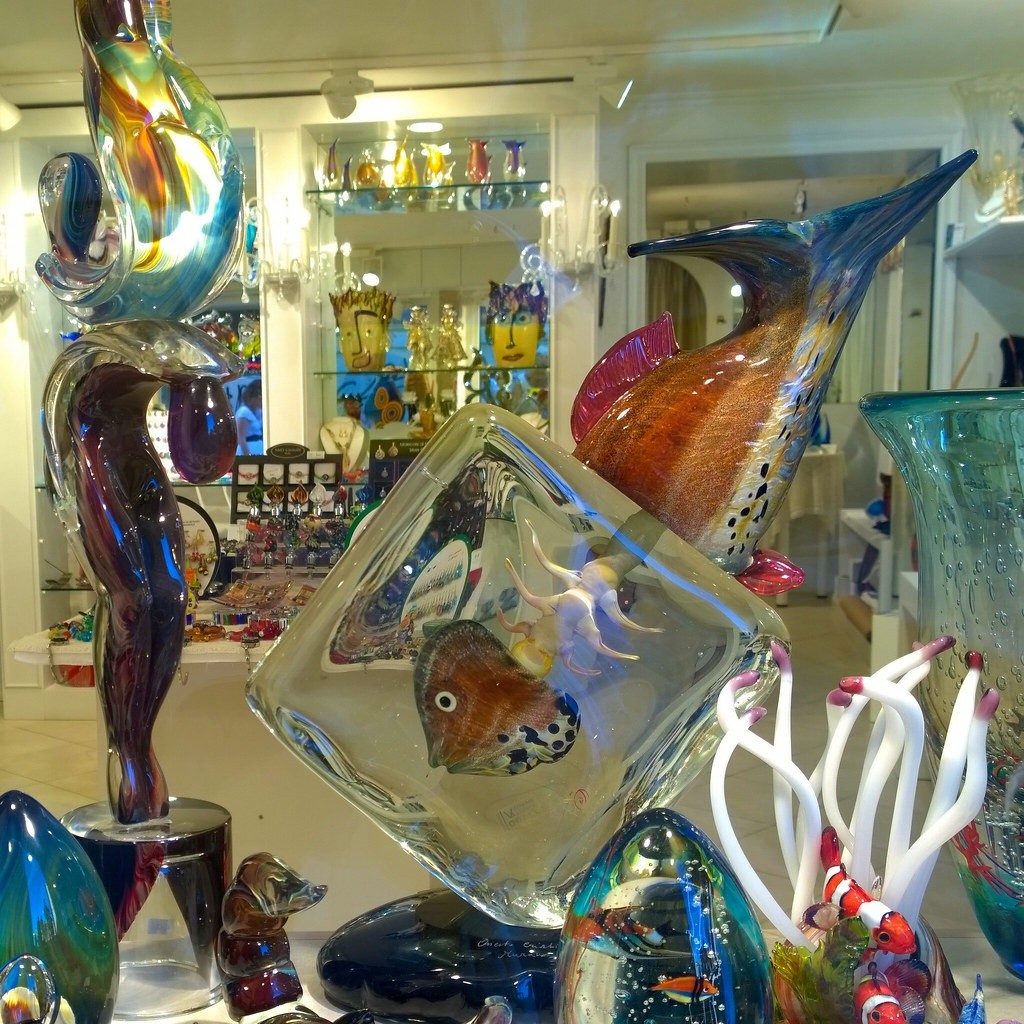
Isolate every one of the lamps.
[569,52,634,110]
[0,94,23,132]
[318,68,375,121]
[520,183,626,300]
[237,190,353,302]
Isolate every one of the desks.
[768,443,844,607]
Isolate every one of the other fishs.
[571,903,721,1006]
[802,823,918,1024]
[415,148,985,777]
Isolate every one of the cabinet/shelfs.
[303,116,557,487]
[833,207,1023,642]
[15,127,270,627]
